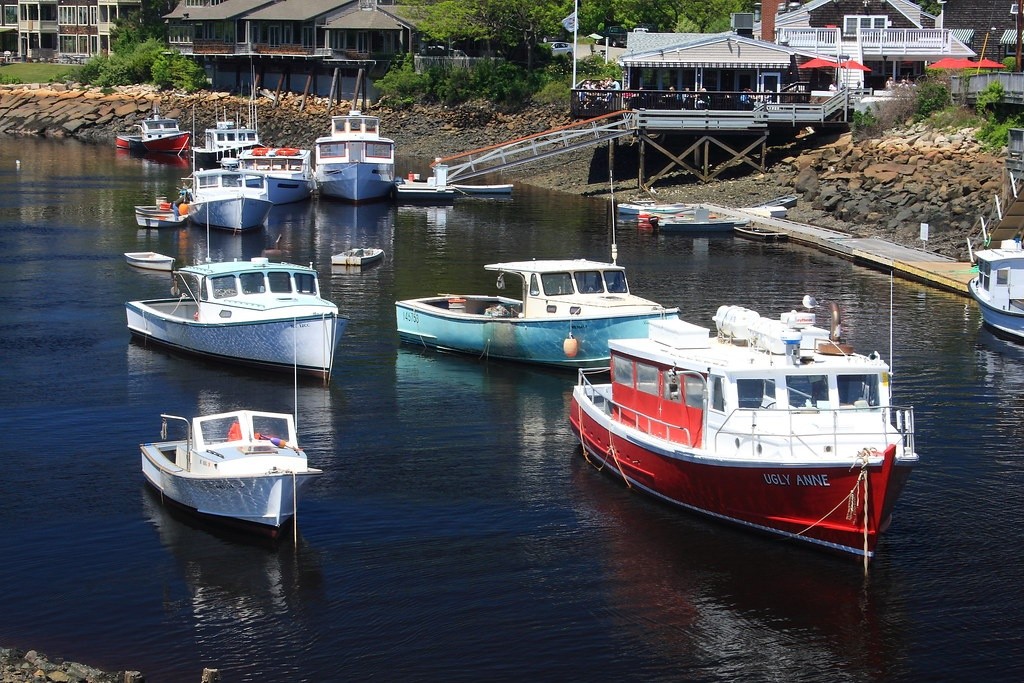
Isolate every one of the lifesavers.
[252,148,268,156]
[276,148,300,156]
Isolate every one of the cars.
[546,41,573,59]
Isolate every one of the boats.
[964,235,1024,341]
[115,105,191,152]
[313,109,395,204]
[179,165,275,234]
[565,306,921,567]
[228,146,315,204]
[393,170,681,368]
[133,202,178,214]
[140,408,325,539]
[124,249,175,271]
[133,214,188,228]
[742,194,798,218]
[123,251,351,381]
[733,226,788,243]
[188,104,267,171]
[329,247,385,267]
[616,200,749,231]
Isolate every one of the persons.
[669,86,711,111]
[738,88,773,105]
[789,80,810,100]
[625,86,647,108]
[854,81,862,94]
[578,77,621,109]
[829,80,838,92]
[887,76,916,90]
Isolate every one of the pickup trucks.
[596,26,628,47]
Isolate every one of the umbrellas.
[928,57,1007,71]
[834,59,873,88]
[799,55,836,88]
